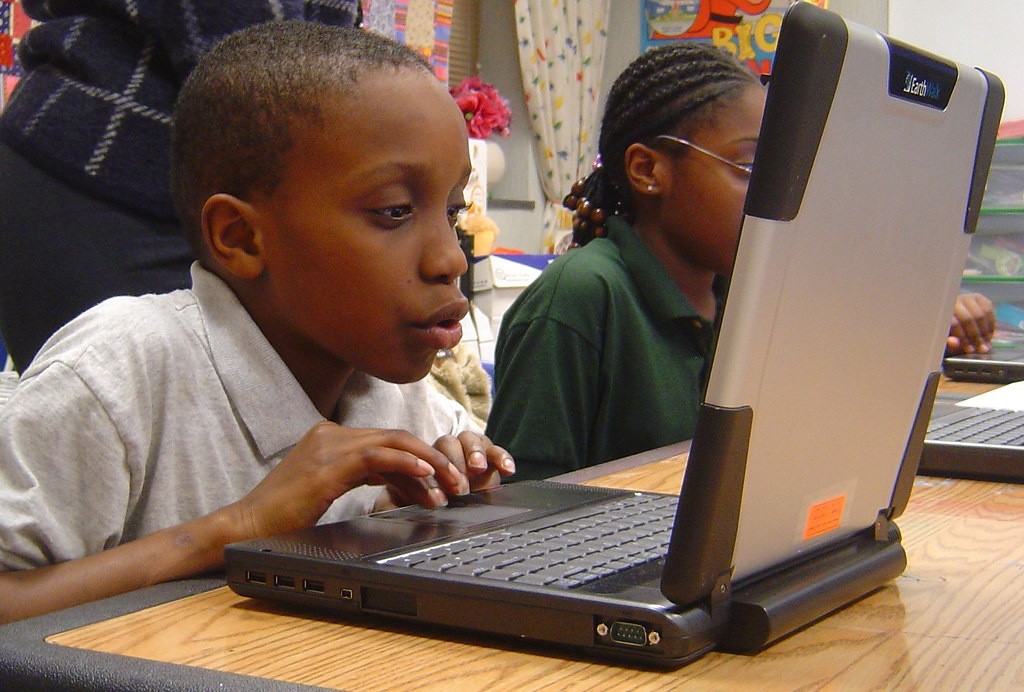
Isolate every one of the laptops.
[915,403,1024,483]
[942,346,1024,382]
[225,1,1006,667]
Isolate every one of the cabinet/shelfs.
[960,137,1024,350]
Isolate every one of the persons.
[484,42,768,485]
[0,22,515,627]
[946,292,997,356]
[0,0,361,376]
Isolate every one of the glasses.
[654,133,753,177]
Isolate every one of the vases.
[463,138,486,215]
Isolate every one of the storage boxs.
[472,255,565,319]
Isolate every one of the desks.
[0,371,1024,692]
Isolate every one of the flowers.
[450,76,513,141]
